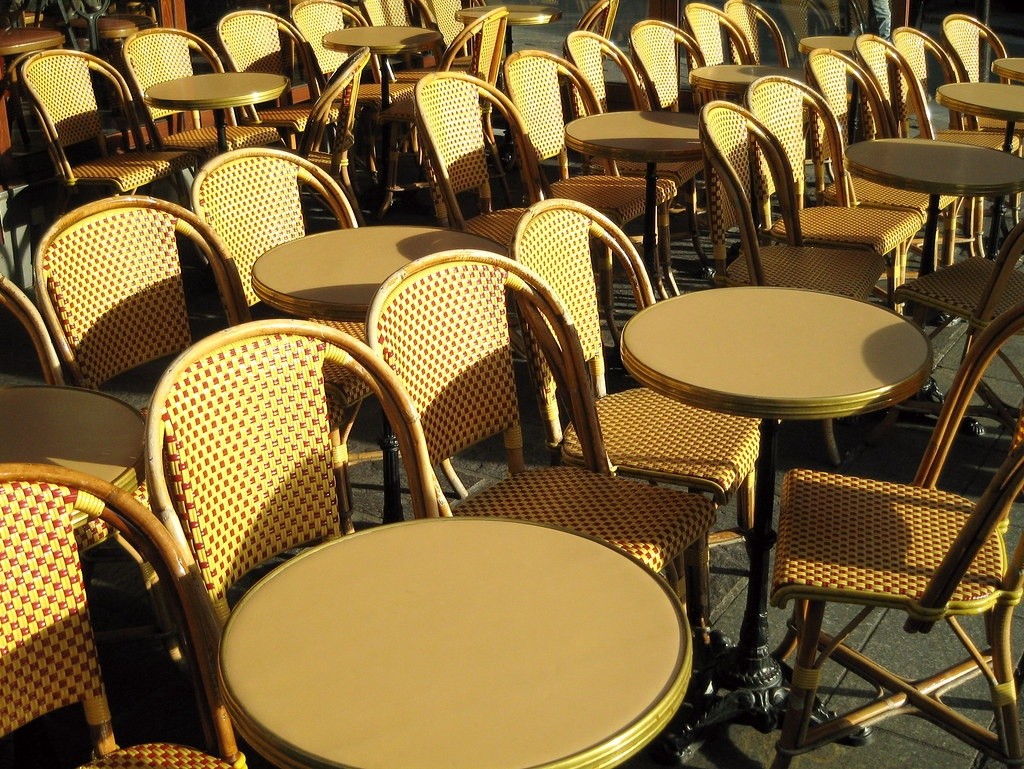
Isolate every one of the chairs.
[0,0,1024,769]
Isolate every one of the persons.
[868,0,892,42]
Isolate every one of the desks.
[69,15,159,117]
[218,517,694,769]
[455,4,563,172]
[799,34,859,144]
[690,64,805,262]
[616,280,935,756]
[322,25,445,225]
[935,82,1024,261]
[562,110,702,292]
[991,57,1024,84]
[0,384,146,769]
[146,71,292,153]
[842,137,1024,439]
[0,28,67,158]
[252,224,509,524]
